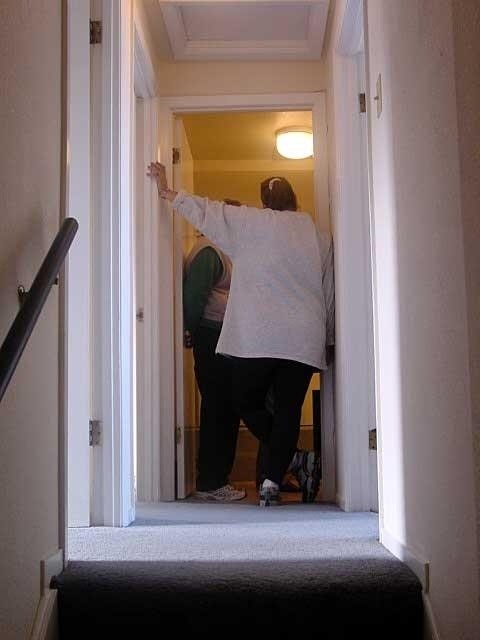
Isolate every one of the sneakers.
[258,480,281,506]
[292,448,320,503]
[193,484,246,502]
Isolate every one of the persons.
[182,199,246,502]
[146,161,334,507]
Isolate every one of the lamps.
[275,127,313,161]
